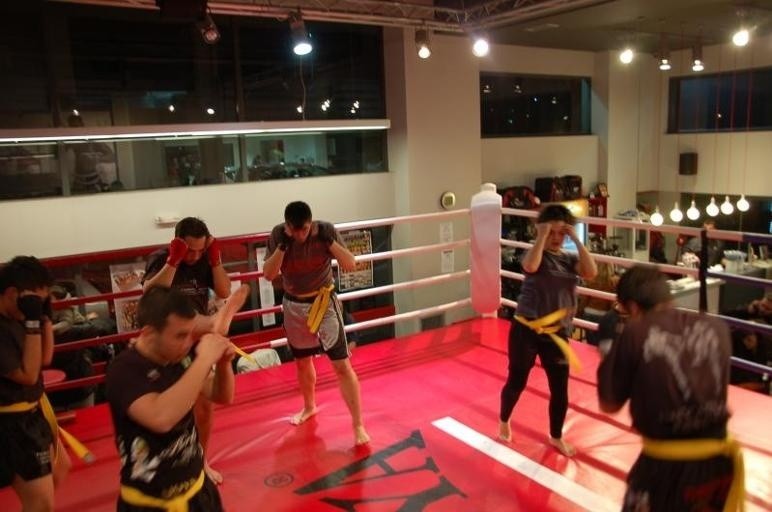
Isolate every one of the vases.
[736,259,745,270]
[724,260,738,274]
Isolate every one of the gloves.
[17,294,42,331]
[277,233,292,250]
[39,294,53,321]
[206,238,221,268]
[317,222,334,246]
[164,239,187,268]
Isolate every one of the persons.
[597,264,746,512]
[166,147,203,187]
[728,324,772,387]
[142,217,231,486]
[65,112,114,195]
[722,295,772,326]
[42,286,98,413]
[0,256,55,512]
[649,230,667,265]
[577,260,622,346]
[106,285,236,512]
[343,312,359,349]
[500,205,598,457]
[681,220,725,268]
[263,202,371,448]
[248,143,313,182]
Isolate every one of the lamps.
[721,44,737,216]
[688,34,705,75]
[669,18,687,223]
[705,23,724,217]
[739,37,759,212]
[656,37,671,69]
[417,22,430,61]
[282,11,313,59]
[194,8,222,43]
[733,8,750,47]
[650,18,665,226]
[687,23,705,221]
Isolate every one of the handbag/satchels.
[500,185,540,209]
[535,176,565,202]
[561,175,582,199]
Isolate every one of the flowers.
[736,252,746,260]
[723,249,738,260]
